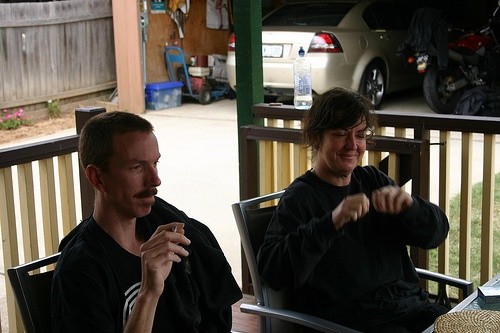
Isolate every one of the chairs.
[6,251,62,333]
[232,188,474,333]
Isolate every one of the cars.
[224,0,445,110]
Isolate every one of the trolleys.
[164,46,236,105]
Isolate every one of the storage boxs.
[145,81,184,111]
[478,286,500,303]
[188,67,209,77]
[208,54,227,66]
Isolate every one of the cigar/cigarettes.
[172,225,178,232]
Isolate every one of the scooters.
[422,1,500,118]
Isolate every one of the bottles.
[190,55,195,67]
[140,0,148,14]
[293,49,313,110]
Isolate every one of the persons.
[51,111,243,333]
[257,87,449,333]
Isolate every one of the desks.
[422,273,500,333]
